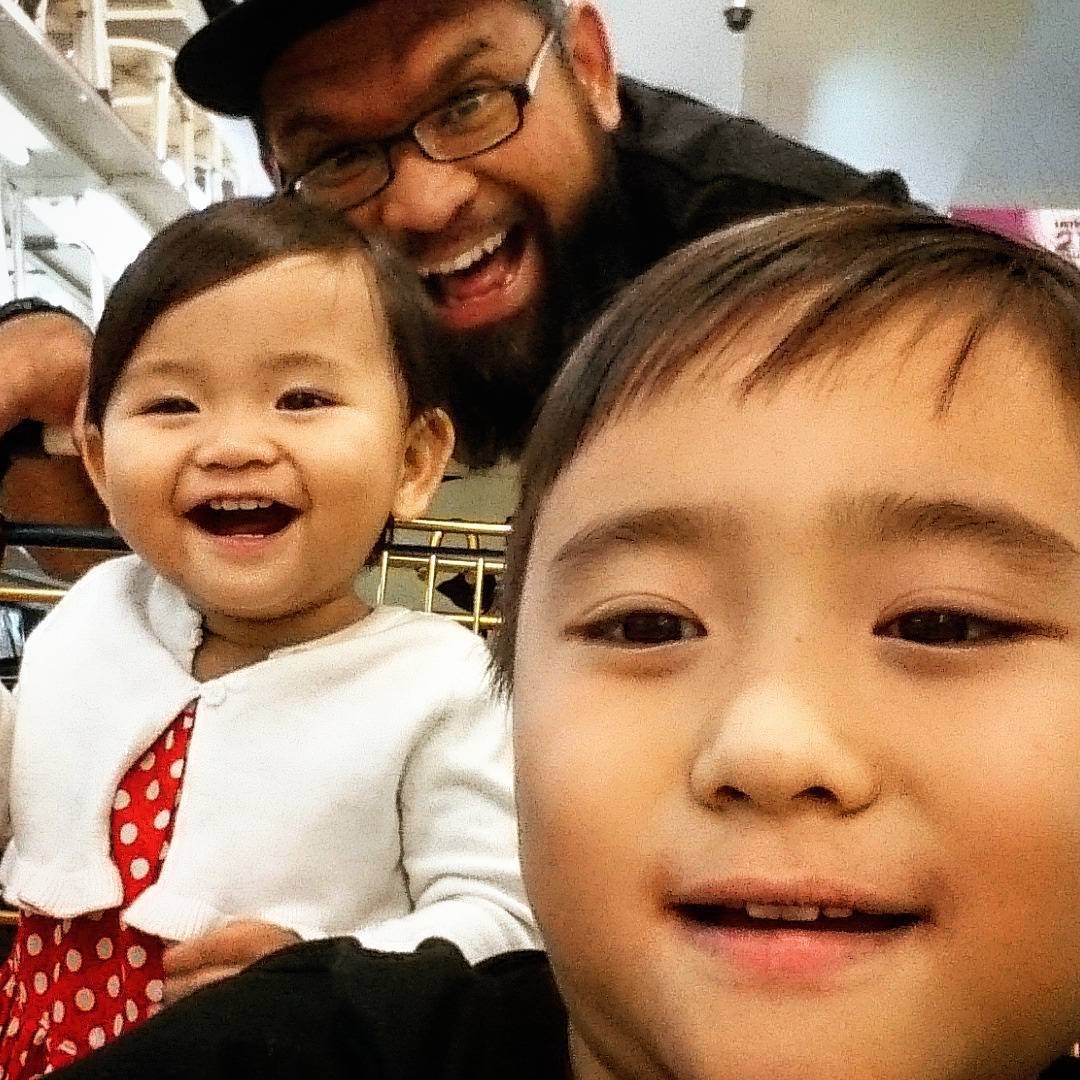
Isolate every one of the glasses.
[261,24,563,213]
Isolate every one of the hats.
[170,0,372,119]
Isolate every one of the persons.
[0,194,549,1080]
[39,202,1080,1079]
[0,1,938,683]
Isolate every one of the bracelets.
[1,298,82,329]
